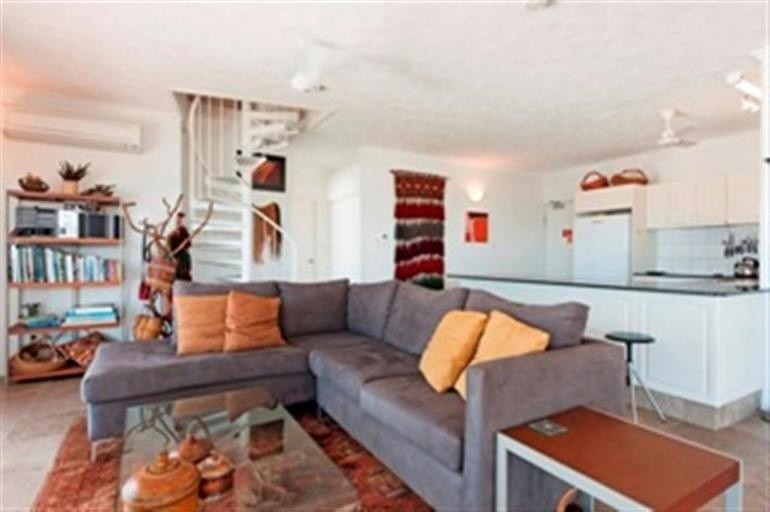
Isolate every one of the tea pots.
[178,437,212,461]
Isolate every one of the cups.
[196,454,235,502]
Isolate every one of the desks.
[495,405,744,512]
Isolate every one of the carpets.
[30,416,432,512]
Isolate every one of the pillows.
[226,289,287,350]
[453,309,549,403]
[418,309,489,394]
[176,296,232,353]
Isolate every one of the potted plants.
[56,158,92,194]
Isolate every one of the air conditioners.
[3,112,144,154]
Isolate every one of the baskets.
[15,335,68,371]
[581,171,609,190]
[611,169,648,185]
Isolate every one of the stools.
[601,322,672,427]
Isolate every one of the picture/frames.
[237,150,287,194]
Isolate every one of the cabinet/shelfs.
[644,172,760,232]
[8,190,122,380]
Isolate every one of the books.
[10,242,123,283]
[64,305,117,325]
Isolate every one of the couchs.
[80,277,629,512]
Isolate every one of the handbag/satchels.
[153,290,172,317]
[145,257,177,289]
[233,460,286,508]
[139,280,151,299]
[133,312,161,342]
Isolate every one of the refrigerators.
[571,208,632,286]
